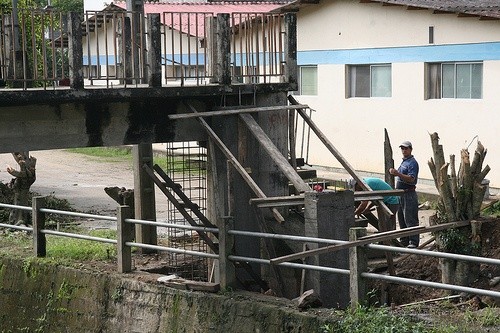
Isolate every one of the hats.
[352,180,357,191]
[399,141,412,147]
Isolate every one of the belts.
[398,185,415,189]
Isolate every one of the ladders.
[142,162,270,294]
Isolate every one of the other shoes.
[407,242,418,247]
[396,242,409,246]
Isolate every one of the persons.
[389,141,419,248]
[352,178,400,257]
